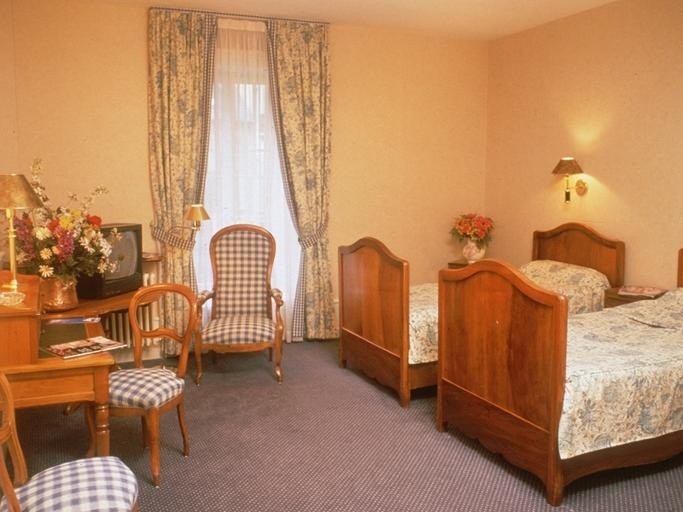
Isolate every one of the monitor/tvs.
[75,223,142,300]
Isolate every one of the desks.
[39,285,167,418]
[0,269,163,459]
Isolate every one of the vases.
[461,238,485,265]
[39,273,77,312]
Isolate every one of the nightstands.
[604,284,669,308]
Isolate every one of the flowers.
[450,212,495,249]
[14,172,126,279]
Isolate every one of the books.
[38,335,128,359]
[47,318,102,325]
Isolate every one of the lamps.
[0,174,43,288]
[551,156,583,203]
[168,204,210,234]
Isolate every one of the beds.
[338,221,626,409]
[436,248,683,507]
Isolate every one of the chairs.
[0,367,139,512]
[193,223,286,382]
[83,282,198,487]
